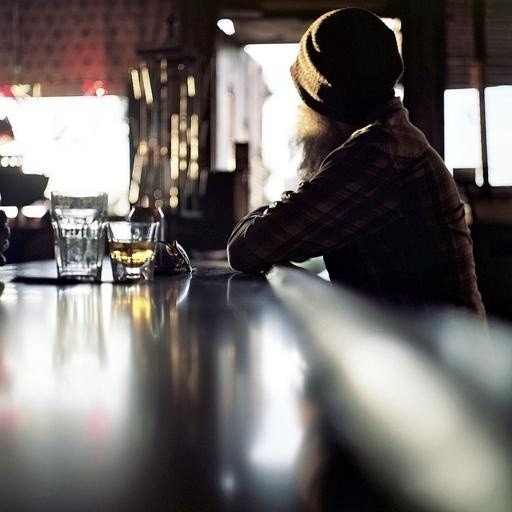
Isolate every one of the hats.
[290,7,404,124]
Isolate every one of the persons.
[227,6,486,318]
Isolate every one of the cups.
[49,187,162,284]
